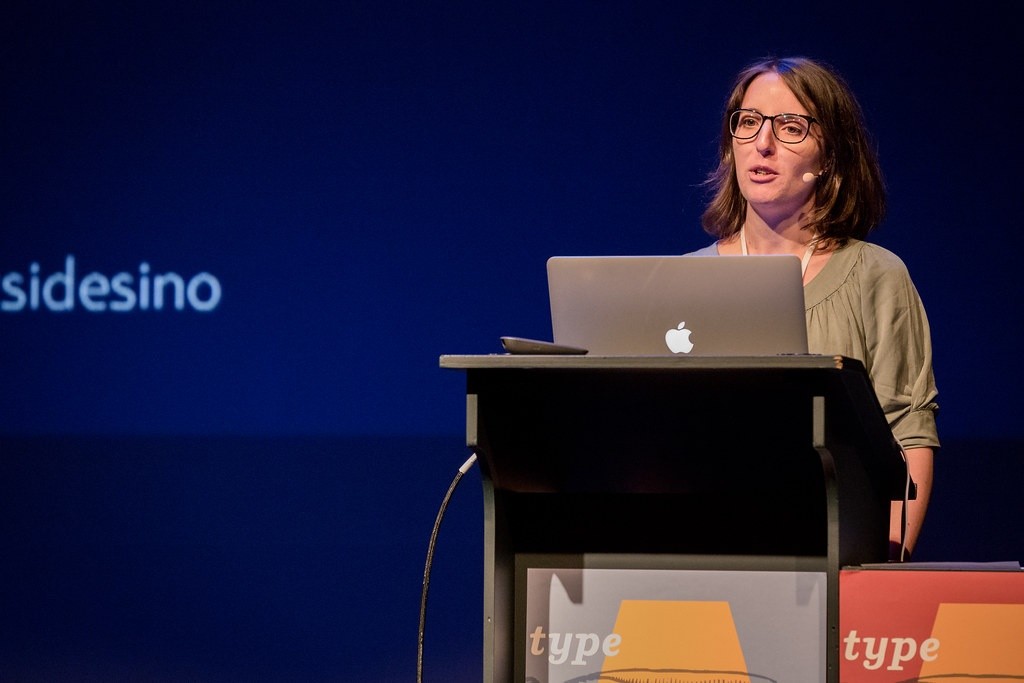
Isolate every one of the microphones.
[803,169,823,183]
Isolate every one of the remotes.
[501,337,587,356]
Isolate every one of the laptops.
[547,255,809,356]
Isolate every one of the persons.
[688,60,940,562]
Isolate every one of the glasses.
[728,107,823,144]
